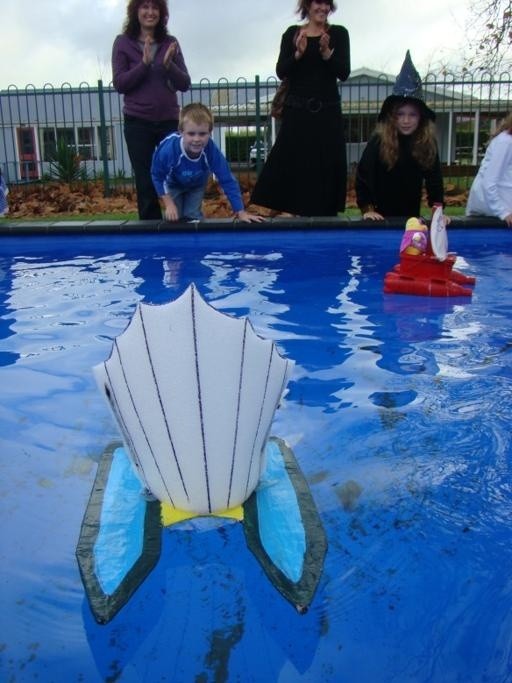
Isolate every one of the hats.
[377,49,436,126]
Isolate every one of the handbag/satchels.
[270,78,288,120]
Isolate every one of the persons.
[111,0,194,220]
[150,99,266,223]
[247,0,352,219]
[463,111,512,227]
[350,47,447,222]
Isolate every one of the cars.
[249,140,269,163]
